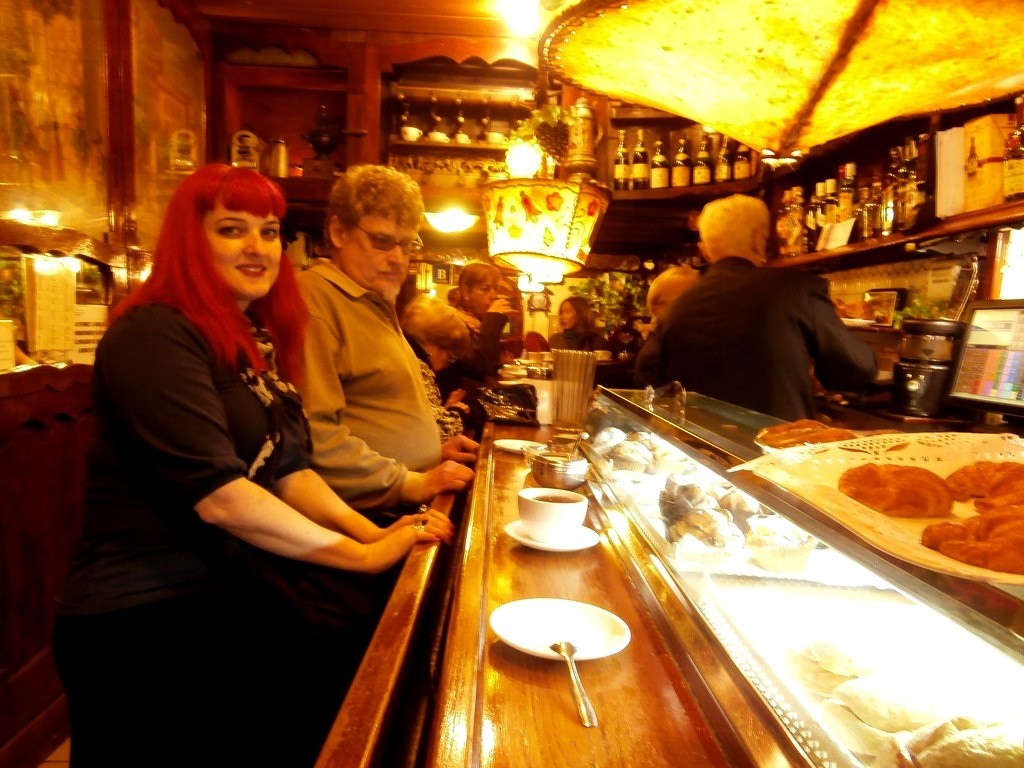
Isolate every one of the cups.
[400,126,423,142]
[485,132,509,144]
[427,132,450,143]
[497,349,555,377]
[456,134,472,144]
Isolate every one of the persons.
[548,296,619,360]
[449,262,515,376]
[632,268,699,390]
[663,193,881,423]
[399,295,471,443]
[295,163,479,511]
[48,166,457,768]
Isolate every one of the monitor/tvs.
[941,298,1024,417]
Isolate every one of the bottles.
[269,139,288,178]
[966,135,977,177]
[1003,127,1024,202]
[612,125,926,259]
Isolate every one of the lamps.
[530,0,1024,164]
[477,179,608,287]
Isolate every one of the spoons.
[550,640,599,728]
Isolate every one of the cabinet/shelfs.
[221,64,1024,266]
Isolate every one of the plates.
[489,597,632,663]
[491,439,547,454]
[504,519,600,552]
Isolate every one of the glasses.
[436,341,459,370]
[349,220,424,257]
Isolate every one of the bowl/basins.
[518,487,588,547]
[533,452,589,490]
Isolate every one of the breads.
[841,456,1024,577]
[593,426,826,575]
[794,629,1023,767]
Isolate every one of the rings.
[416,526,425,536]
[413,514,418,521]
[419,503,430,514]
